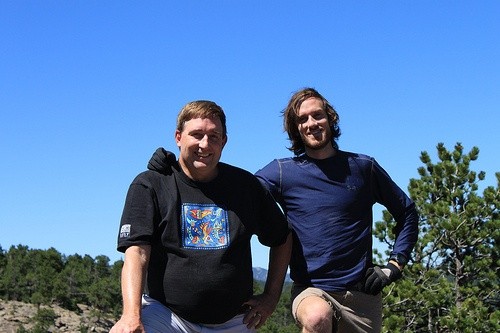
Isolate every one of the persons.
[147,86,419,333]
[108,100,296,333]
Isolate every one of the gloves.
[361,263,402,296]
[147,147,176,171]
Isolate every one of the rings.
[257,313,262,317]
